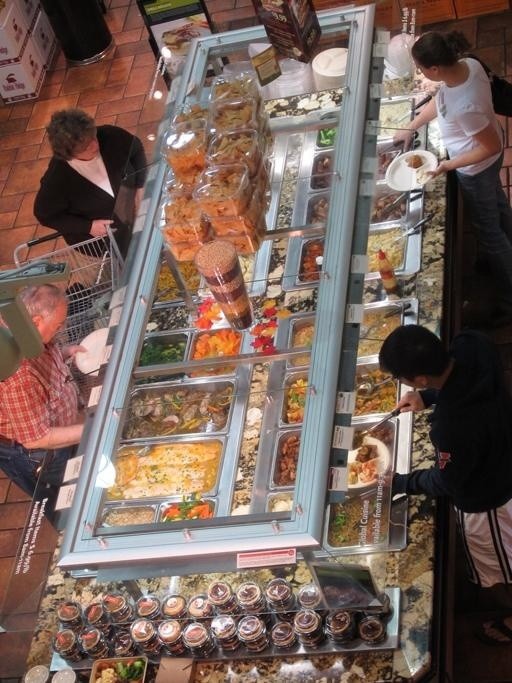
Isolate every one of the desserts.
[55,578,390,662]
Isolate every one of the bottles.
[163,595,187,617]
[297,584,323,608]
[111,632,142,655]
[266,581,296,618]
[236,581,268,620]
[376,247,398,292]
[82,604,112,635]
[268,621,298,649]
[182,623,216,657]
[209,614,239,652]
[323,610,356,646]
[52,629,80,660]
[54,602,85,631]
[101,593,136,629]
[293,610,323,648]
[188,595,214,618]
[133,595,163,621]
[129,617,163,655]
[209,581,241,620]
[156,619,186,656]
[235,616,271,652]
[76,627,111,660]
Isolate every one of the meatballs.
[410,156,422,168]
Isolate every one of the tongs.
[380,130,419,170]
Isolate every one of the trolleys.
[10,217,132,409]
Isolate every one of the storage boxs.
[2,0,57,106]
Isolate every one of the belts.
[0,435,19,447]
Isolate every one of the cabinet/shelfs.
[1,1,464,682]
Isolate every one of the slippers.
[474,616,512,644]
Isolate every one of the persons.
[2,283,91,531]
[377,323,512,644]
[392,30,512,329]
[30,108,149,332]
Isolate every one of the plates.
[385,150,439,192]
[311,47,348,91]
[348,435,392,489]
[75,326,110,377]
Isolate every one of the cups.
[194,240,254,333]
